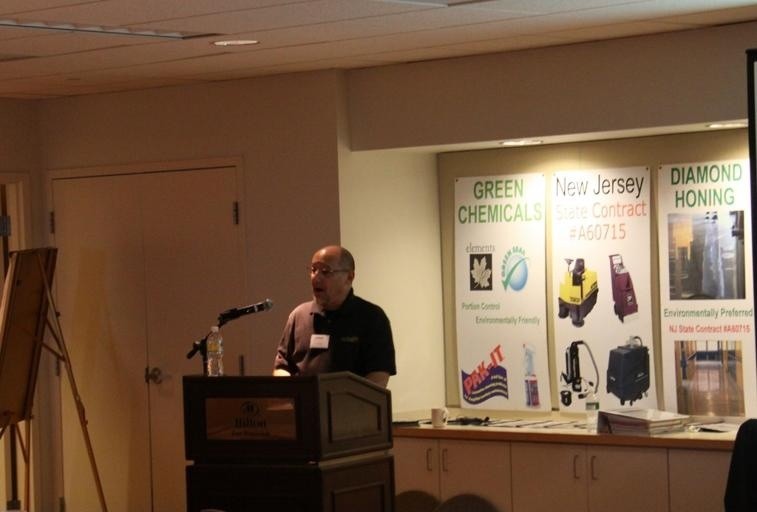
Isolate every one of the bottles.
[206,326,224,377]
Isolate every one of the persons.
[273,243,398,388]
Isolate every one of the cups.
[432,408,449,427]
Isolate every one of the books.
[596,407,692,437]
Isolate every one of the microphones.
[231,297,275,316]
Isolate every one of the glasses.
[310,265,349,275]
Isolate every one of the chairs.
[723,419,757,512]
